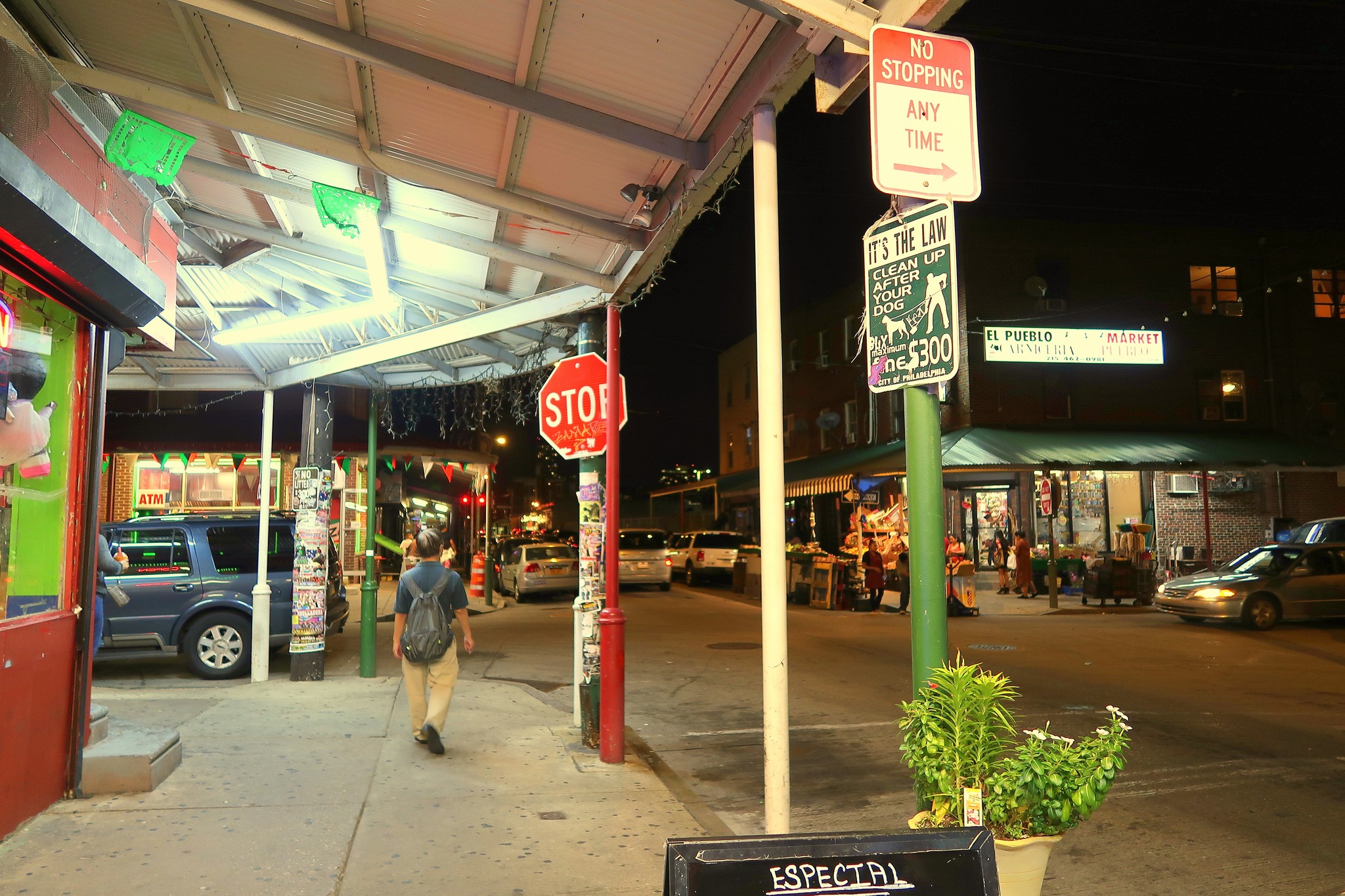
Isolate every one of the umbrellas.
[1144,501,1154,547]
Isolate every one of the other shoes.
[871,611,877,615]
[900,610,905,615]
[1030,592,1039,598]
[1018,594,1029,598]
[876,609,884,614]
[996,587,1009,595]
[423,725,445,755]
[414,735,427,744]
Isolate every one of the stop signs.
[538,352,628,460]
[1040,478,1052,516]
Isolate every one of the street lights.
[477,436,505,553]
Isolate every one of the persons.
[399,530,420,558]
[1012,531,1038,598]
[393,529,474,754]
[94,524,129,656]
[862,540,885,615]
[984,511,1004,566]
[465,539,475,553]
[491,541,500,588]
[988,529,1011,594]
[895,552,910,614]
[944,533,965,565]
[789,529,804,545]
[439,526,456,568]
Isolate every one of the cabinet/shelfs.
[836,525,907,587]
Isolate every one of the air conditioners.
[1164,472,1199,495]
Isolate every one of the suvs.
[99,513,349,680]
[619,528,670,592]
[670,531,740,587]
[1263,517,1345,563]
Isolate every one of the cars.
[1153,544,1345,632]
[476,532,579,603]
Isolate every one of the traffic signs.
[871,23,981,204]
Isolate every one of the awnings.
[784,473,852,497]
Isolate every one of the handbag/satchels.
[993,550,1004,568]
[1006,551,1017,570]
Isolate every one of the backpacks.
[400,570,455,662]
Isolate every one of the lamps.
[617,179,666,228]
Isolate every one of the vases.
[902,809,1063,896]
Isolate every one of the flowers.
[892,646,1138,839]
